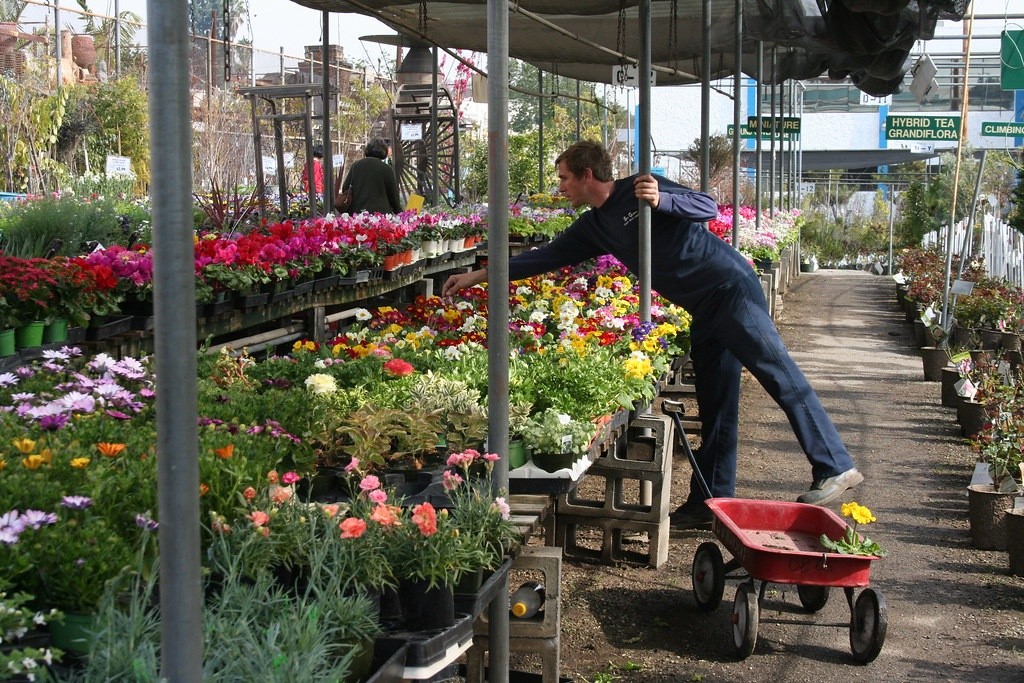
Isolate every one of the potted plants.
[800,212,892,275]
[897,244,1024,577]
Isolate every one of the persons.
[341,137,401,215]
[301,145,325,195]
[440,138,864,529]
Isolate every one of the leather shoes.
[669,509,713,528]
[796,468,863,506]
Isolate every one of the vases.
[404,251,411,263]
[16,321,45,347]
[753,259,772,269]
[464,236,475,248]
[394,252,399,265]
[411,248,421,260]
[423,240,438,257]
[436,239,443,253]
[403,579,455,627]
[508,439,526,471]
[45,317,69,342]
[532,449,573,473]
[458,238,466,249]
[448,239,459,250]
[398,252,407,265]
[383,254,395,271]
[475,235,482,243]
[442,238,449,251]
[0,328,15,357]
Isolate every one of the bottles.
[510,581,545,619]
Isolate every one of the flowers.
[707,206,803,276]
[0,175,691,683]
[820,502,889,558]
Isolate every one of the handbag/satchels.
[334,187,352,213]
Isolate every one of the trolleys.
[661,397,890,665]
